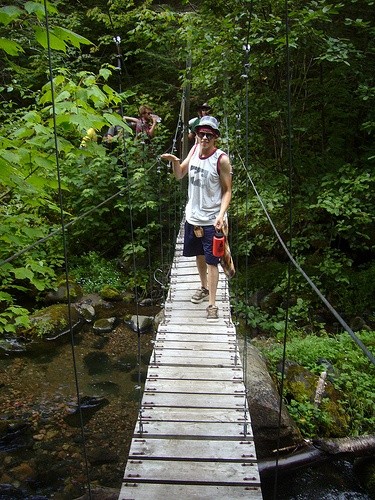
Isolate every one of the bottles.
[149,113,162,122]
[213,230,224,257]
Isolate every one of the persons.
[104,111,127,172]
[186,102,215,142]
[135,104,159,141]
[159,116,233,320]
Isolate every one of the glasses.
[145,112,153,115]
[196,132,216,139]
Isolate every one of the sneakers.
[190,287,209,304]
[205,305,219,322]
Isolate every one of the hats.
[192,116,220,135]
[197,102,213,114]
